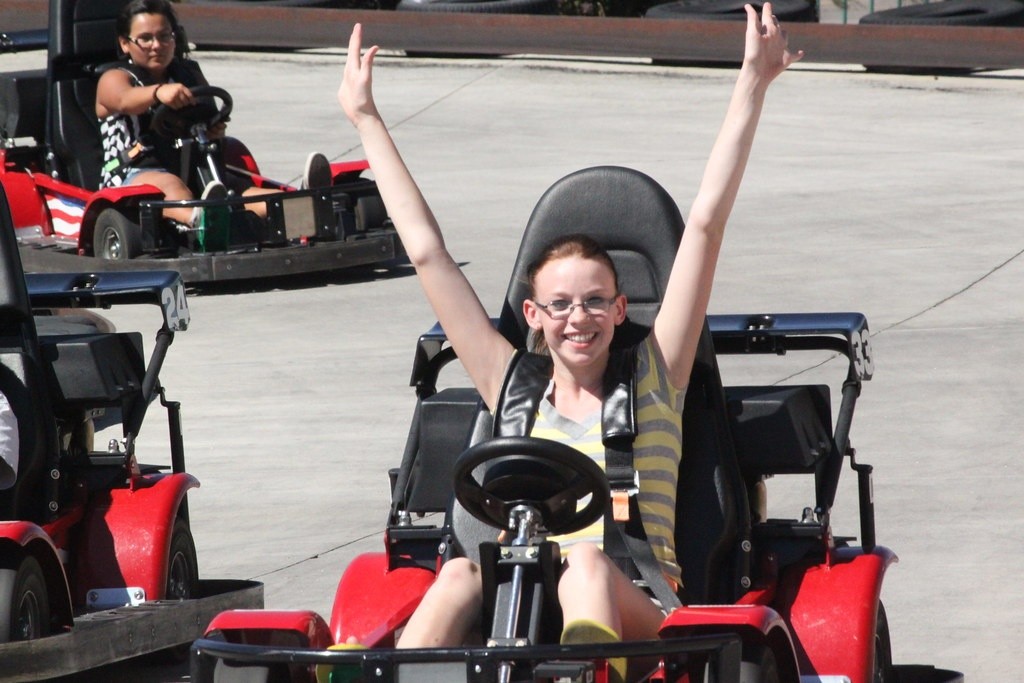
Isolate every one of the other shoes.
[190,181,232,252]
[300,153,334,240]
[316,644,368,683]
[559,621,628,683]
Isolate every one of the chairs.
[45,0,244,200]
[445,166,753,606]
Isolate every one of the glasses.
[124,31,175,49]
[535,295,618,320]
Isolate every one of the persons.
[338,1,804,683]
[95,1,334,252]
[0,391,19,489]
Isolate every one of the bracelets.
[153,83,168,105]
